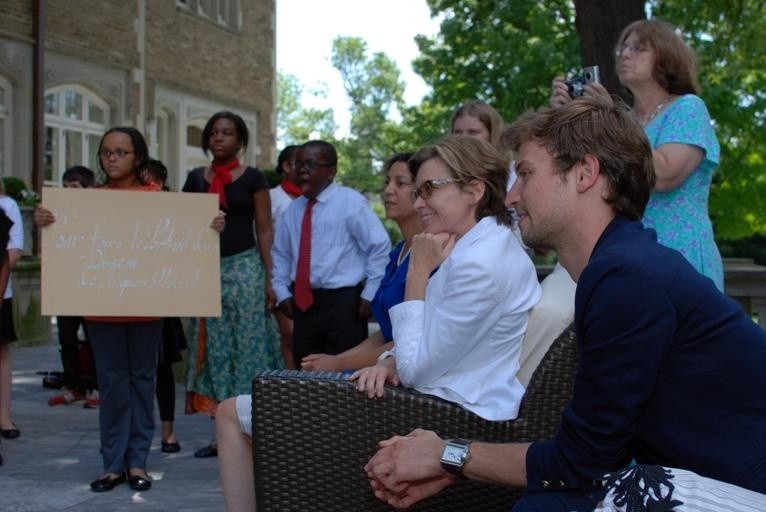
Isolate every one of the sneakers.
[48,388,99,408]
[127,469,152,491]
[90,473,126,493]
[195,444,217,458]
[161,441,181,454]
[0,422,20,440]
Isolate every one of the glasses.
[291,160,331,171]
[410,176,463,204]
[614,43,650,57]
[99,150,135,160]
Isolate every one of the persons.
[297,153,441,379]
[216,135,545,512]
[547,19,727,303]
[364,97,766,512]
[451,102,538,264]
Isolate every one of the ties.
[293,198,318,314]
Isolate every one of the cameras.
[583,65,601,85]
[563,79,584,98]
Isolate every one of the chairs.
[250,319,582,512]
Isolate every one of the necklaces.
[631,93,673,128]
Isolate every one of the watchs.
[438,439,483,485]
